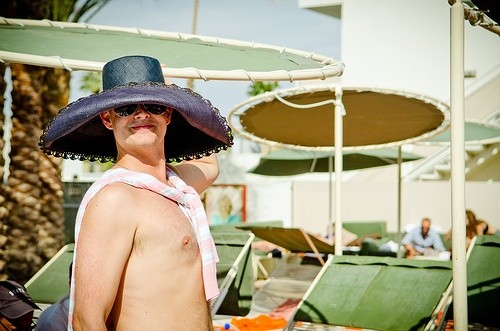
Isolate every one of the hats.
[0,280,43,319]
[39,55,234,163]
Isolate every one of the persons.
[465,210,495,240]
[39,55,234,331]
[402,218,445,259]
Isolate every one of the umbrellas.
[247,145,427,246]
[0,17,345,81]
[227,85,450,255]
[420,119,500,146]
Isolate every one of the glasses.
[113,101,166,117]
[0,319,37,331]
